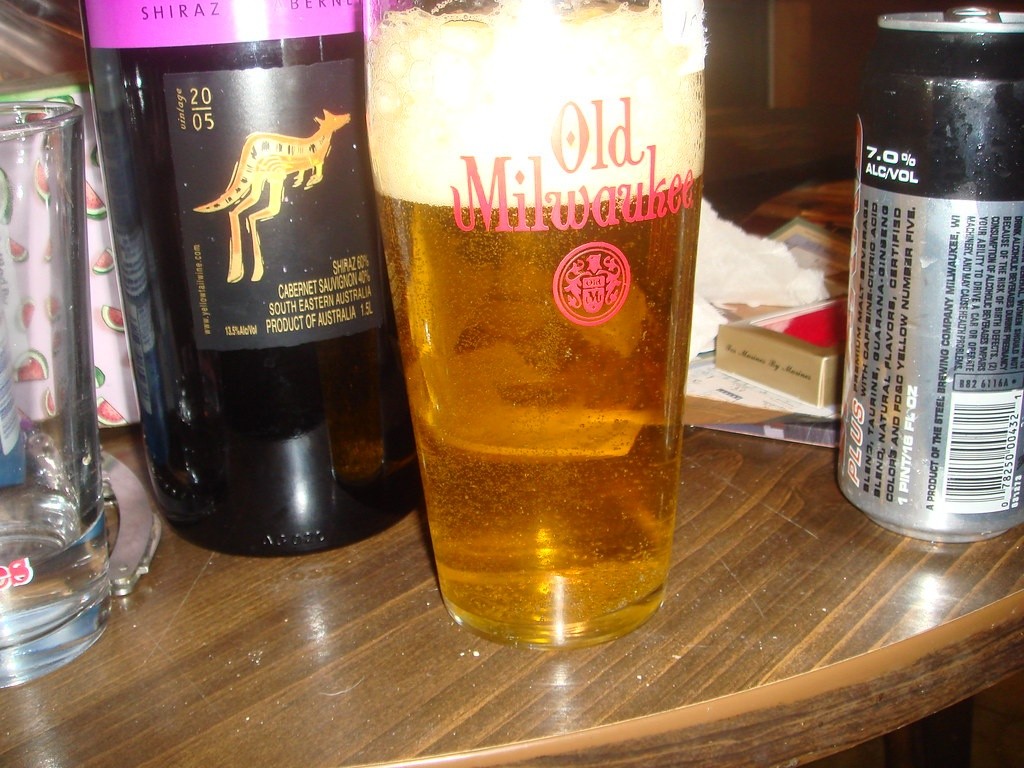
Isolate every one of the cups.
[0,100,110,689]
[351,0,708,649]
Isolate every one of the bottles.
[76,0,422,558]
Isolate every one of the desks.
[1,417,1024,768]
